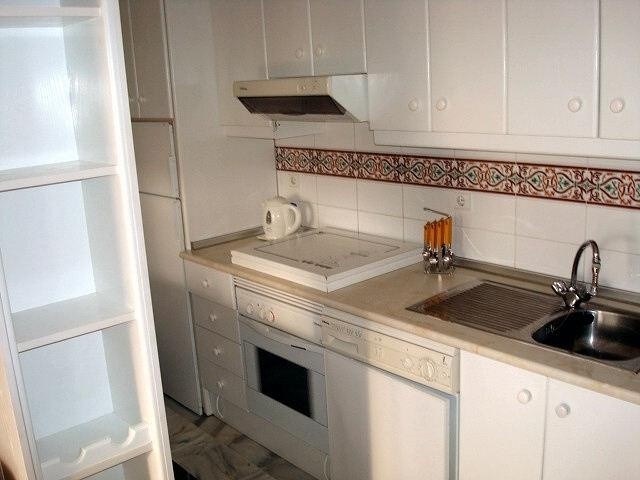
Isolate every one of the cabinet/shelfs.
[364,1,507,152]
[209,0,326,141]
[116,0,279,123]
[505,1,640,172]
[183,260,250,415]
[261,1,368,77]
[131,120,278,416]
[1,0,175,479]
[459,350,640,480]
[323,348,458,480]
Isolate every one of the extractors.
[231,69,366,132]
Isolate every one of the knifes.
[438,222,442,272]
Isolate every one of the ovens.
[231,290,330,451]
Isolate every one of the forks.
[428,223,436,272]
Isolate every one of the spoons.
[444,216,456,271]
[422,224,430,261]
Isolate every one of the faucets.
[551,240,601,308]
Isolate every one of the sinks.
[531,303,640,361]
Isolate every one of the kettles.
[260,194,302,240]
